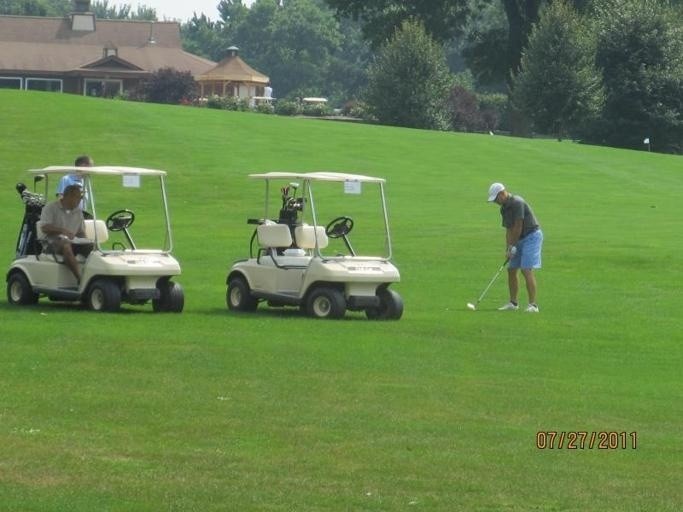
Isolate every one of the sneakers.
[524,305,539,314]
[497,301,519,312]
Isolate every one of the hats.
[486,182,504,202]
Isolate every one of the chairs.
[35,221,62,262]
[294,226,328,265]
[83,220,108,250]
[257,224,294,265]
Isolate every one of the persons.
[487,182,545,311]
[263,81,274,100]
[39,186,86,285]
[55,156,93,217]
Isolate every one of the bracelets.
[506,248,511,252]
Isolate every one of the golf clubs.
[466,254,511,310]
[16,175,47,211]
[281,179,307,217]
